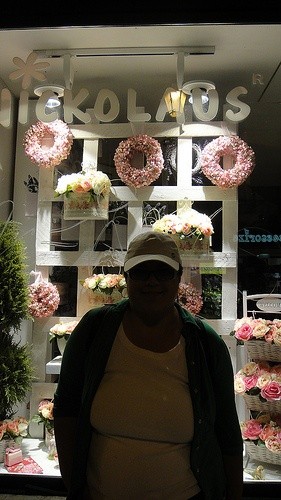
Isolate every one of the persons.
[52,231,244,500]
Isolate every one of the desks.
[0,438,281,500]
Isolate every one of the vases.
[45,428,55,446]
[0,440,16,462]
[57,337,67,356]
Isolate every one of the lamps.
[182,79,215,104]
[32,84,66,108]
[164,88,187,118]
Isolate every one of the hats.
[124,234,182,272]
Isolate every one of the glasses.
[127,267,177,282]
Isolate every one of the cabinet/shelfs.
[243,290,281,460]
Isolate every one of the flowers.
[32,400,54,435]
[0,416,29,441]
[234,316,281,345]
[200,136,256,189]
[240,414,281,480]
[79,273,129,307]
[234,359,281,402]
[152,209,214,241]
[28,281,60,321]
[48,322,79,343]
[178,282,203,315]
[23,120,165,198]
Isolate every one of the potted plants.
[0,220,40,441]
